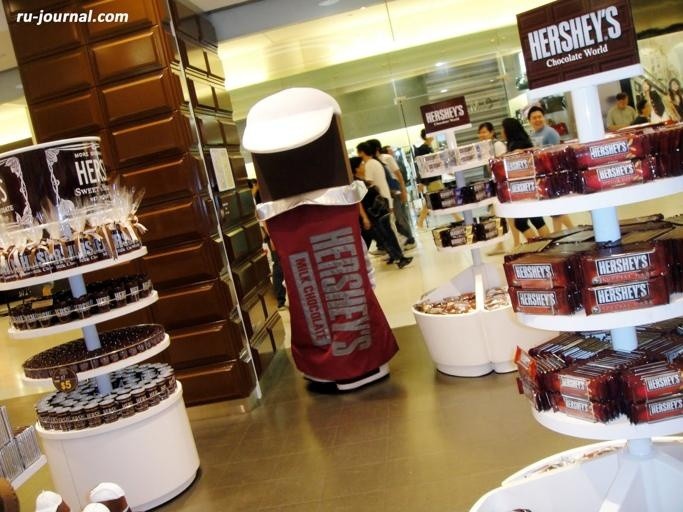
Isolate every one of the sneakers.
[398,257,413,269]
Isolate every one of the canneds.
[33,361,177,431]
[0,136,111,234]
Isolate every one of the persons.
[233,77,683,315]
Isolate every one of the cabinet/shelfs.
[0,135,200,511]
